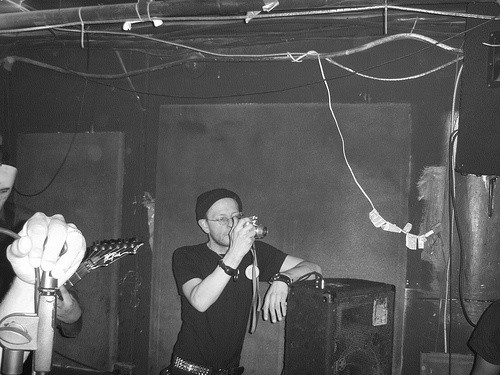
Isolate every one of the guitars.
[62,237,144,289]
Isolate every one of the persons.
[469,299,500,375]
[171,188,322,375]
[0,144,86,375]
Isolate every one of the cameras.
[243,216,268,239]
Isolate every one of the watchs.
[270,272,291,287]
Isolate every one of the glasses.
[209,212,244,225]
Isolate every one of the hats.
[194,187,242,221]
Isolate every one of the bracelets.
[218,260,236,277]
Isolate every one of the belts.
[175,357,244,375]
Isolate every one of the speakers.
[284,278,396,375]
[455,3,500,176]
